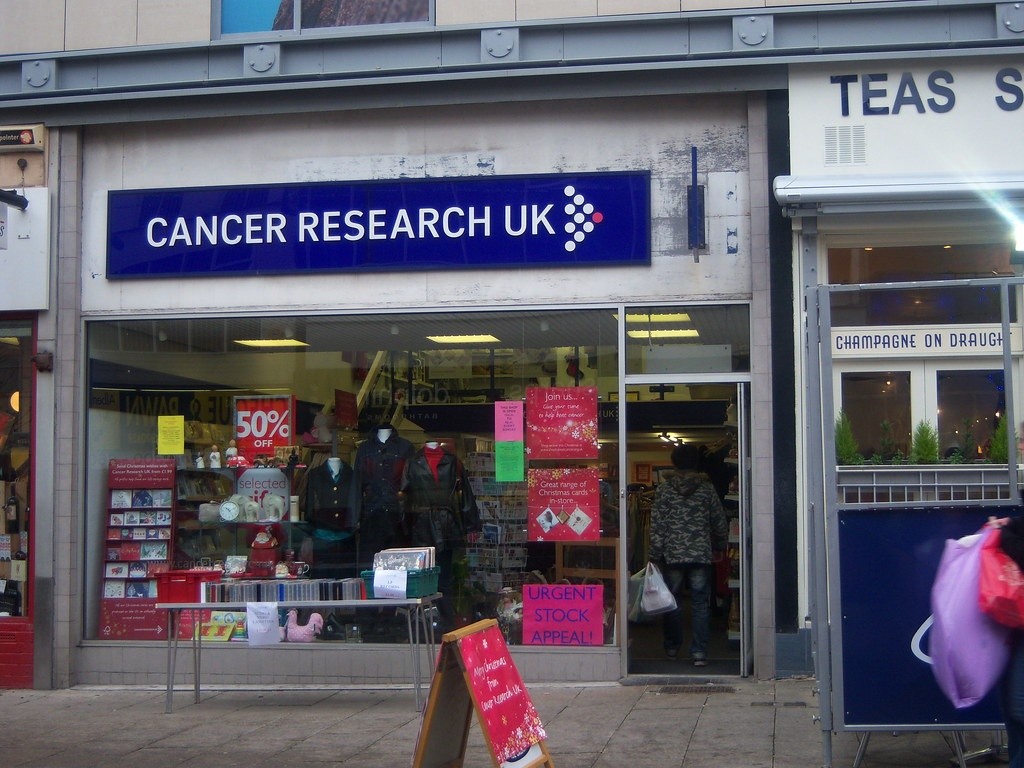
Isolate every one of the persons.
[399,441,482,632]
[987,423,1024,768]
[305,457,353,620]
[345,425,416,636]
[252,524,278,549]
[196,439,237,469]
[648,444,728,666]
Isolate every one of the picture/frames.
[608,391,640,401]
[632,462,653,486]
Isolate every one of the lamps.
[660,434,683,447]
[0,189,29,211]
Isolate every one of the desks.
[155,594,442,712]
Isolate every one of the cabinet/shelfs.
[724,421,739,640]
[174,467,226,560]
[556,538,621,646]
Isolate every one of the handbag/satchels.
[933,519,1024,710]
[628,561,678,623]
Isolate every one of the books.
[462,452,527,593]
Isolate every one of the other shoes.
[667,648,676,656]
[694,658,708,666]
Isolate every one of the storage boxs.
[153,569,223,603]
[362,566,440,598]
